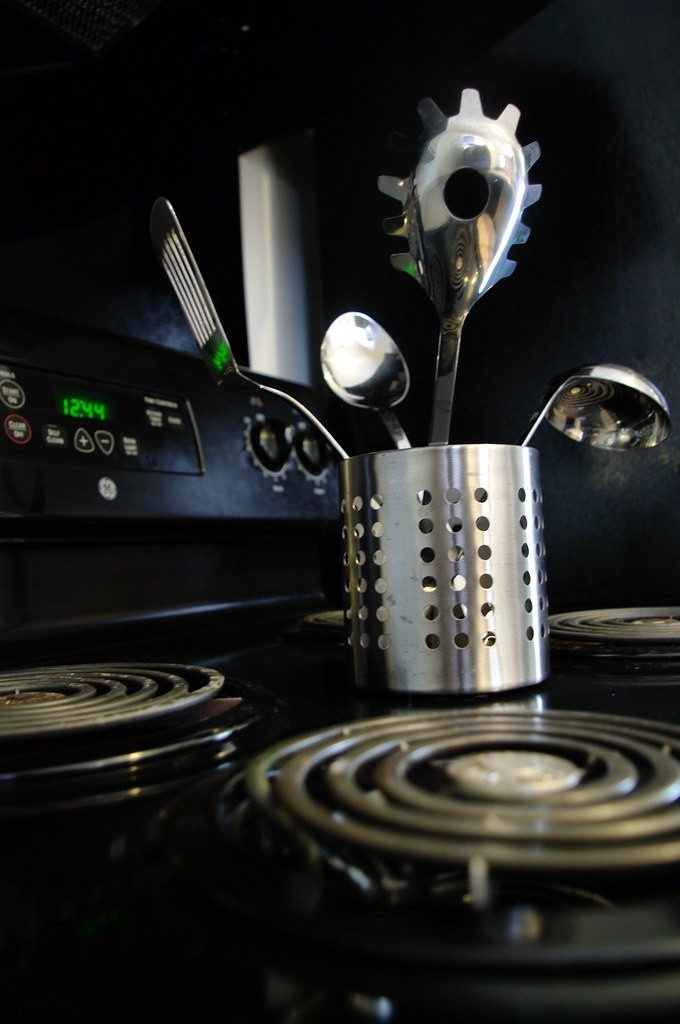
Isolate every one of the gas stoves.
[0,307,680,1024]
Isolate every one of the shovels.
[143,195,349,459]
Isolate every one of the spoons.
[522,364,671,457]
[315,310,422,455]
